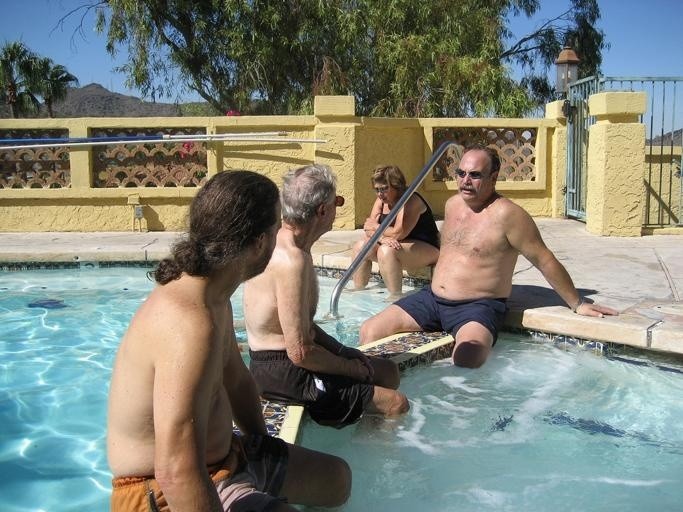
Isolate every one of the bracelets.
[572,297,585,313]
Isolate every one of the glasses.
[326,196,344,207]
[455,169,482,179]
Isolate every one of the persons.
[243,165,410,430]
[352,165,441,295]
[106,169,352,512]
[360,146,619,368]
